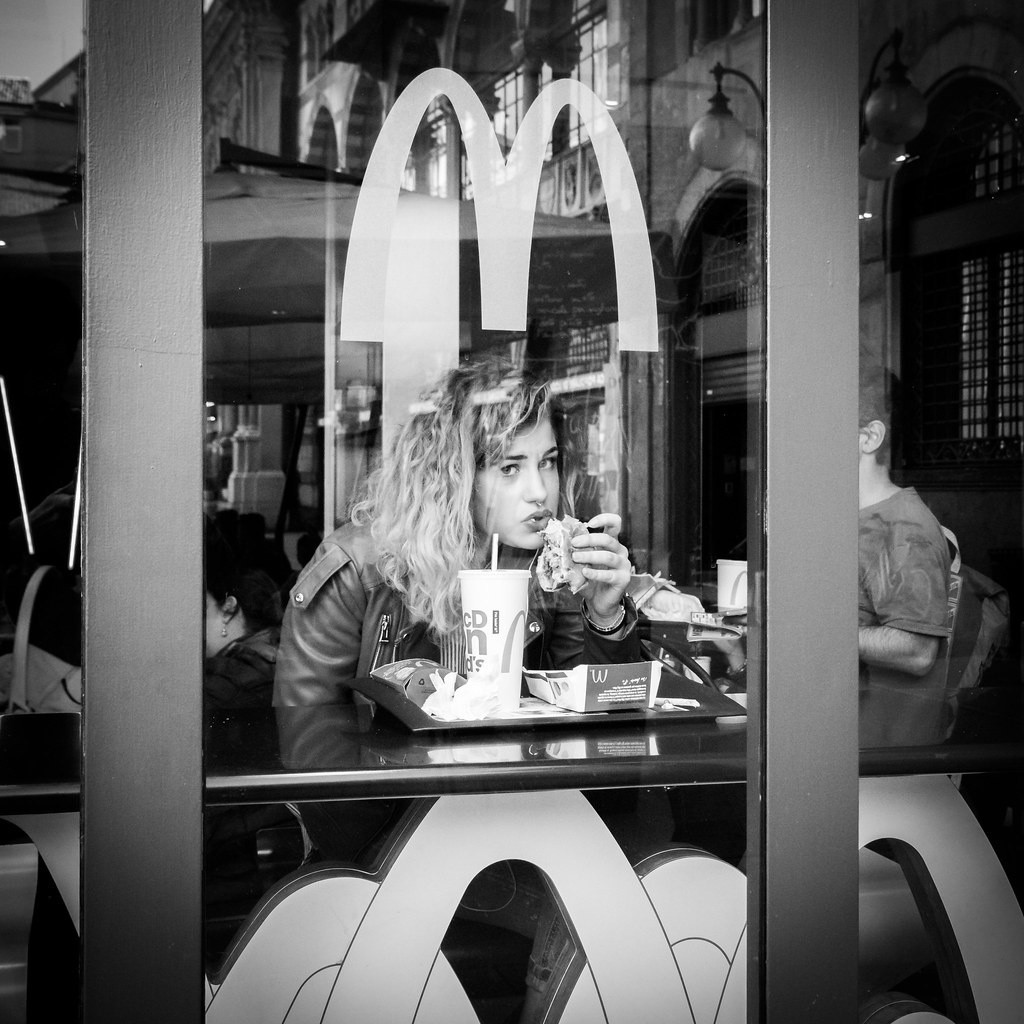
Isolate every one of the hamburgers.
[536,511,595,594]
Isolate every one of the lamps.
[688,61,764,172]
[859,29,929,181]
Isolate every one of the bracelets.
[582,599,625,632]
[586,615,626,636]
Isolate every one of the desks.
[0,682,1024,1024]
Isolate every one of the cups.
[717,559,748,612]
[458,568,531,715]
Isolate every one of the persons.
[270,358,639,704]
[670,363,949,867]
[202,509,323,710]
